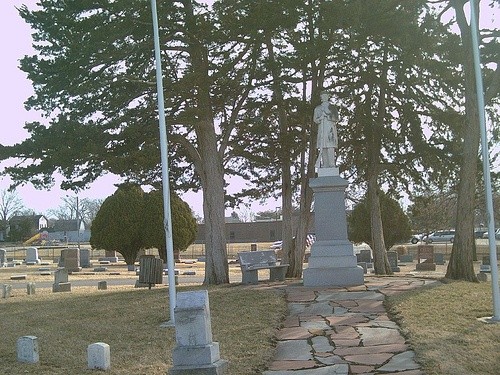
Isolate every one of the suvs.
[422,230,455,243]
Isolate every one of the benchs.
[235,249,290,285]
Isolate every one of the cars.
[474,227,500,239]
[409,230,420,244]
